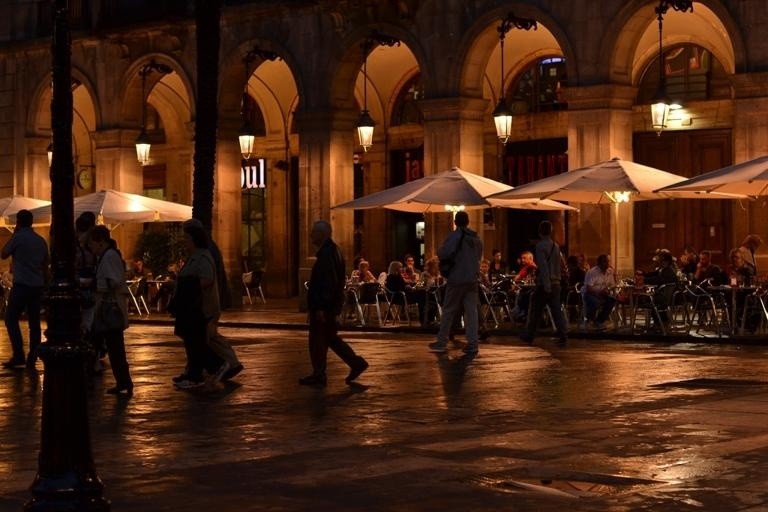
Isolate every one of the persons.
[297,220,369,386]
[519,222,569,349]
[559,246,755,330]
[346,257,444,306]
[0,210,245,395]
[480,251,536,287]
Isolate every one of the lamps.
[492,18,537,146]
[136,64,172,167]
[47,144,55,167]
[353,35,400,153]
[238,51,277,160]
[650,0,693,137]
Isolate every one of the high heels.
[107,383,133,394]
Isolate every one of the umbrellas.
[0,196,51,215]
[480,157,756,331]
[0,190,195,229]
[329,166,580,257]
[651,156,768,196]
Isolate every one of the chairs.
[124,274,175,318]
[344,271,587,327]
[241,267,268,304]
[607,278,768,338]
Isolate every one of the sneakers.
[172,363,244,389]
[2,357,26,367]
[461,342,479,353]
[428,342,448,350]
[25,359,36,369]
[591,322,607,330]
[584,316,592,328]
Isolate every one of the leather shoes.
[519,334,533,342]
[559,335,569,344]
[345,359,369,382]
[298,375,328,388]
[477,331,491,340]
[449,334,455,341]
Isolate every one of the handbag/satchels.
[438,253,456,279]
[96,301,124,335]
